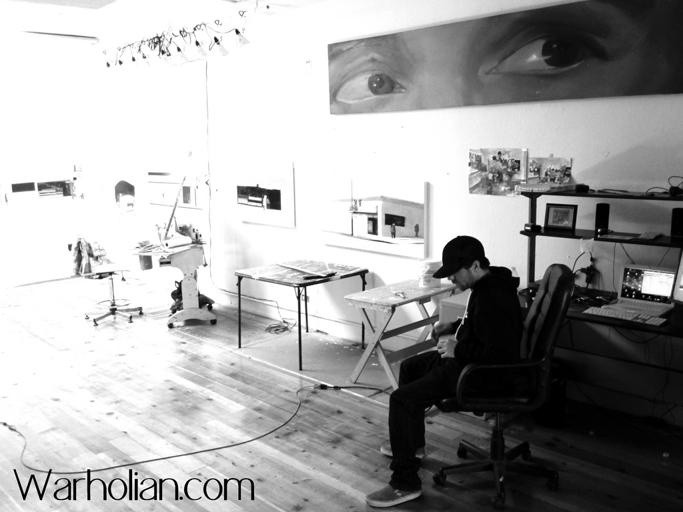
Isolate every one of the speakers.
[594,203,610,238]
[670,208,683,244]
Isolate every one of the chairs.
[72,231,143,326]
[430,263,575,511]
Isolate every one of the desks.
[234,259,459,392]
[519,285,682,398]
[141,227,220,326]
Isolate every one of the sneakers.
[380,441,425,460]
[366,483,422,508]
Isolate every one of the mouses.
[671,312,683,327]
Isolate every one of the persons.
[365,236,523,507]
[328,0,683,114]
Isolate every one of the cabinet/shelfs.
[519,186,683,289]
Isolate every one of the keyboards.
[582,307,667,326]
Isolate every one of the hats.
[432,236,485,279]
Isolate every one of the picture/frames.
[544,203,578,233]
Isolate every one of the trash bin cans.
[532,360,583,429]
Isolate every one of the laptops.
[600,264,677,317]
